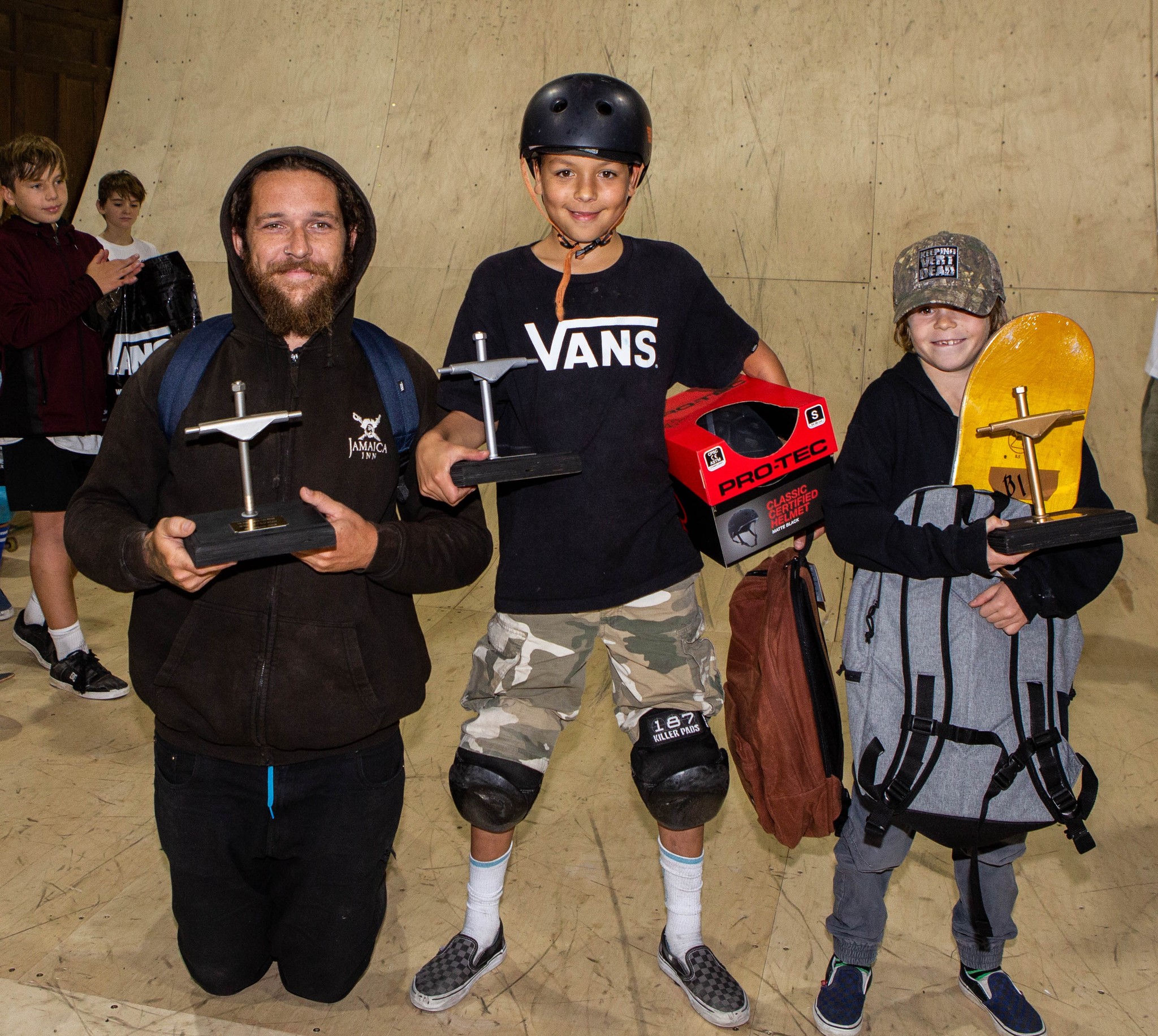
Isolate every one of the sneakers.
[958,962,1048,1036]
[48,650,130,700]
[409,919,507,1012]
[12,609,58,670]
[813,953,873,1036]
[656,926,750,1029]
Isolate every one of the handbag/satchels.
[78,250,205,425]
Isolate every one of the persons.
[813,232,1122,1036]
[95,170,158,265]
[64,145,495,1003]
[409,74,826,1028]
[0,134,145,699]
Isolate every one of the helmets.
[518,71,654,171]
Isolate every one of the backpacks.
[722,525,850,850]
[833,480,1101,939]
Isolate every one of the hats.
[890,232,1006,322]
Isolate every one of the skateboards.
[950,312,1094,510]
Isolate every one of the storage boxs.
[659,370,839,571]
